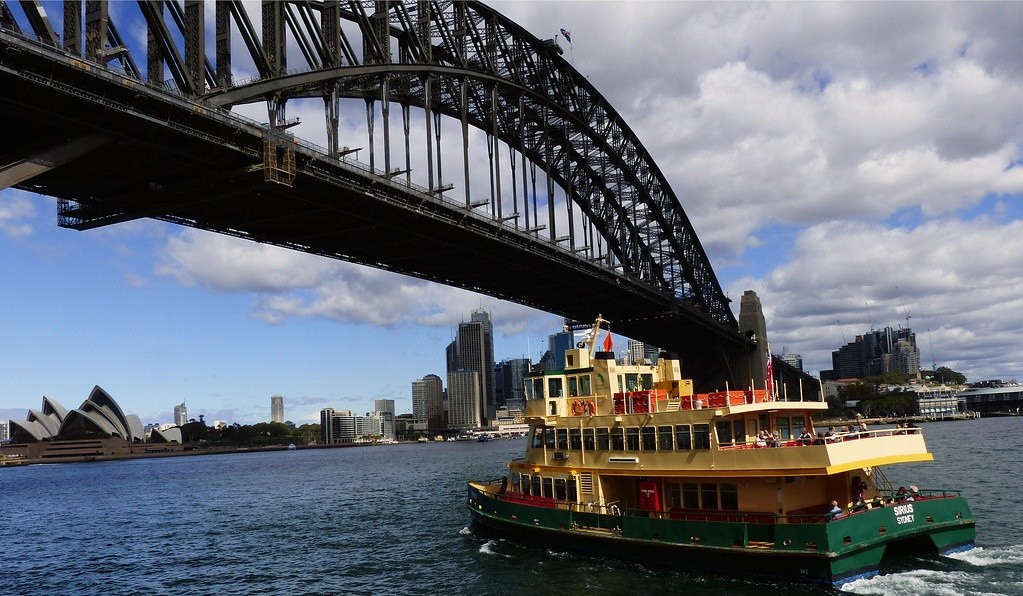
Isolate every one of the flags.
[603,332,613,352]
[560,29,571,43]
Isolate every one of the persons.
[753,419,918,449]
[494,477,508,498]
[854,486,921,511]
[851,475,868,501]
[828,500,842,522]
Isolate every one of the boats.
[462,312,975,591]
